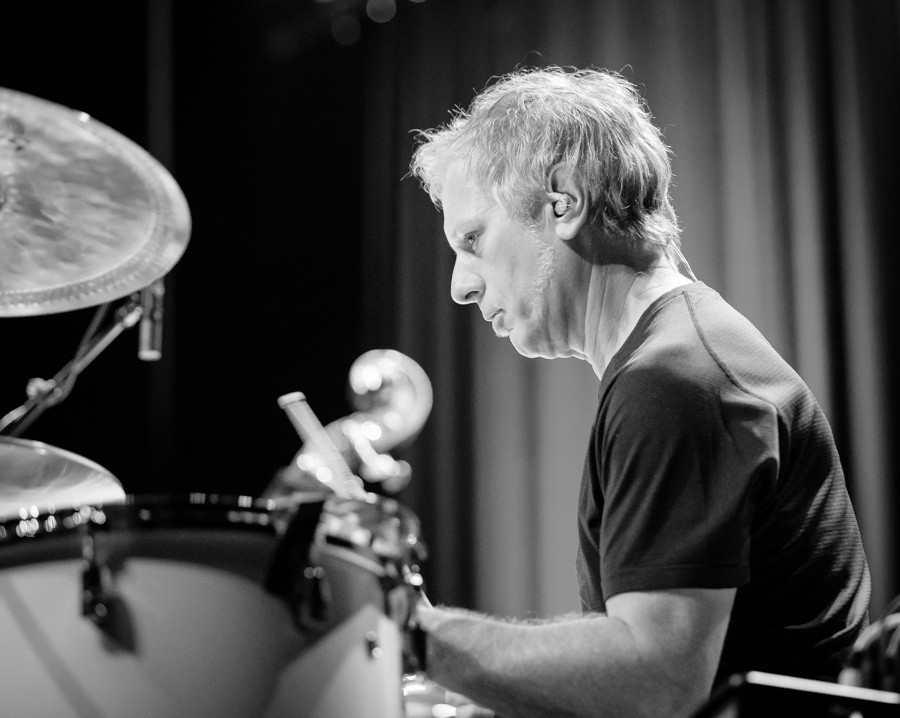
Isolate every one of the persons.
[403,66,871,718]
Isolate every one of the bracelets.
[408,606,440,675]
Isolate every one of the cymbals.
[0,436,126,521]
[0,87,192,317]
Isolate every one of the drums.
[0,492,427,718]
